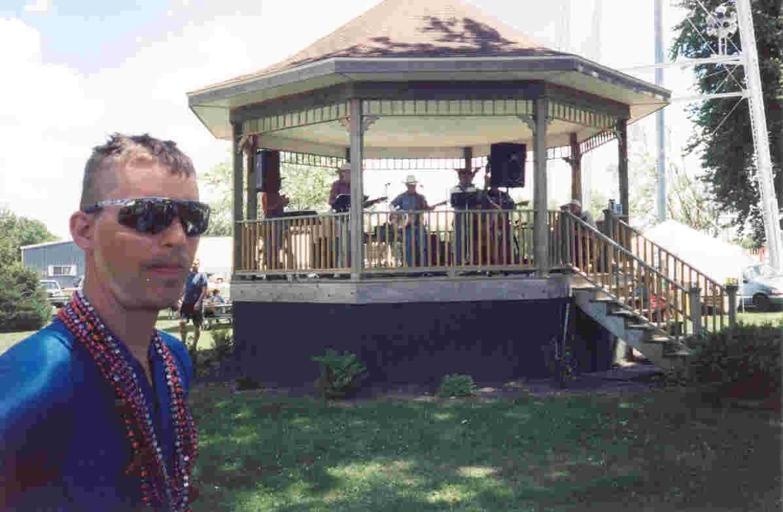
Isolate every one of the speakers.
[254,149,279,193]
[488,141,526,188]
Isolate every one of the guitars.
[389,201,447,229]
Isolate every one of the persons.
[452,166,483,269]
[261,189,288,274]
[1,131,207,512]
[479,174,517,267]
[563,199,598,270]
[162,256,229,354]
[325,164,369,279]
[390,175,436,270]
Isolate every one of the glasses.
[82,196,211,236]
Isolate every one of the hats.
[404,175,418,185]
[568,199,580,206]
[336,165,351,172]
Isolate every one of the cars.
[699,261,783,310]
[37,280,65,308]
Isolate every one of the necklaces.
[40,283,211,507]
